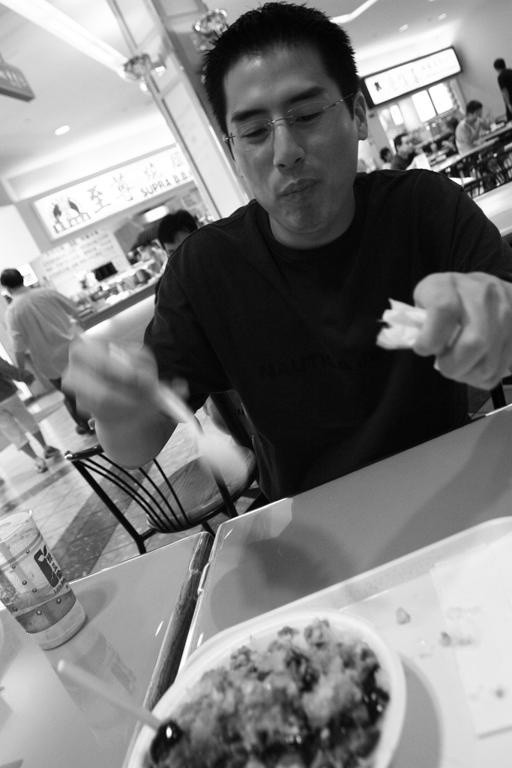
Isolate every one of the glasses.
[221,97,344,149]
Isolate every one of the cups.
[0,508,88,653]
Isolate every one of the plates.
[127,607,408,768]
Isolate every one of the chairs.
[60,441,258,557]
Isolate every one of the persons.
[442,117,459,155]
[378,144,393,171]
[0,356,60,475]
[389,132,417,171]
[0,268,95,437]
[156,209,197,259]
[455,97,499,193]
[52,0,512,513]
[491,56,511,124]
